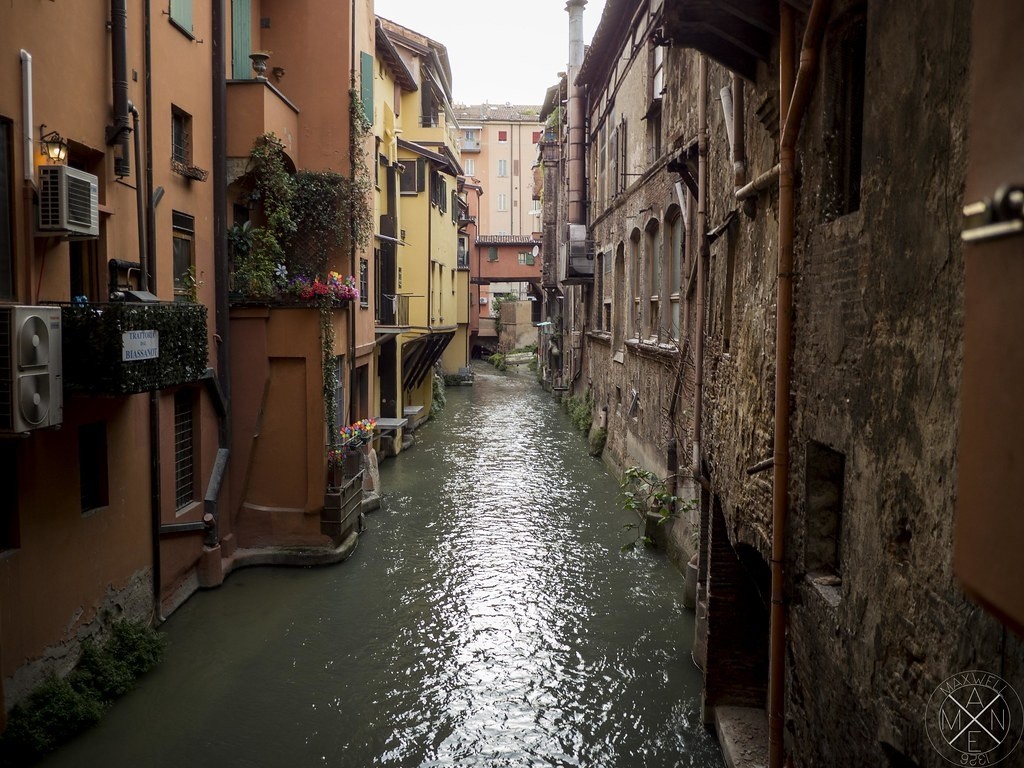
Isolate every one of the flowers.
[340,418,376,435]
[274,263,357,307]
[327,448,347,469]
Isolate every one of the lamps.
[40,124,70,164]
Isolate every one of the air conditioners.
[0,305,63,433]
[479,297,488,305]
[38,164,99,236]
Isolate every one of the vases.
[350,435,372,451]
[329,468,342,486]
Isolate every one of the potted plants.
[249,49,273,76]
